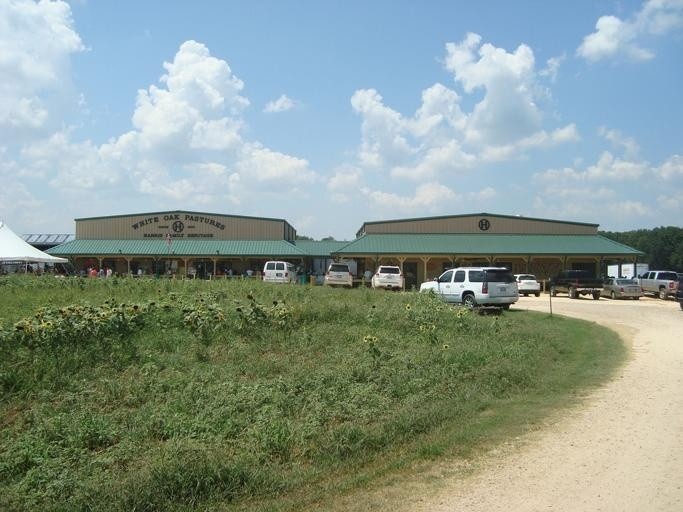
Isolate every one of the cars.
[599,276,645,300]
[674,276,682,311]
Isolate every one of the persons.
[1,267,8,282]
[295,264,316,282]
[87,266,113,278]
[137,264,143,275]
[167,268,172,278]
[188,264,202,279]
[364,267,373,289]
[224,265,233,276]
[19,265,54,275]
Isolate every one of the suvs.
[417,264,521,312]
[324,261,354,288]
[0,264,36,279]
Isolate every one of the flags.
[167,234,174,252]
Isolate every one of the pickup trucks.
[632,270,682,300]
[548,268,605,300]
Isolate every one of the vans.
[371,264,404,291]
[260,260,298,285]
[513,273,542,297]
